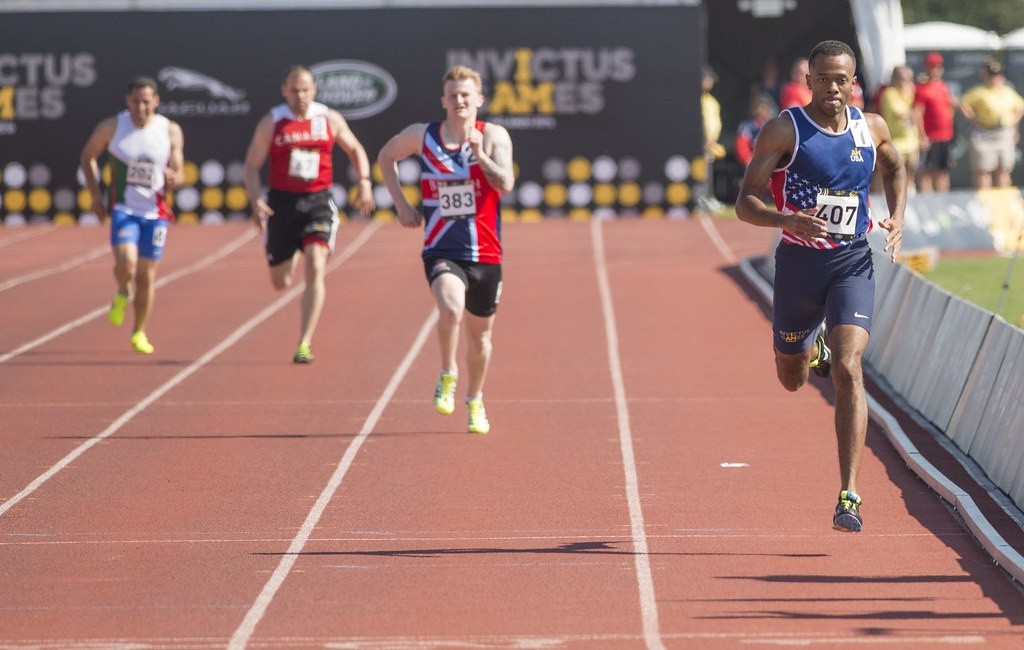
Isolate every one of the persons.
[79,76,185,350]
[699,40,1024,531]
[378,64,515,435]
[241,68,371,366]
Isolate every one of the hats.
[924,52,943,69]
[984,59,1003,74]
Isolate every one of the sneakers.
[434,371,457,415]
[130,332,155,354]
[809,336,832,377]
[833,490,863,532]
[109,293,129,326]
[465,396,489,433]
[294,345,312,362]
